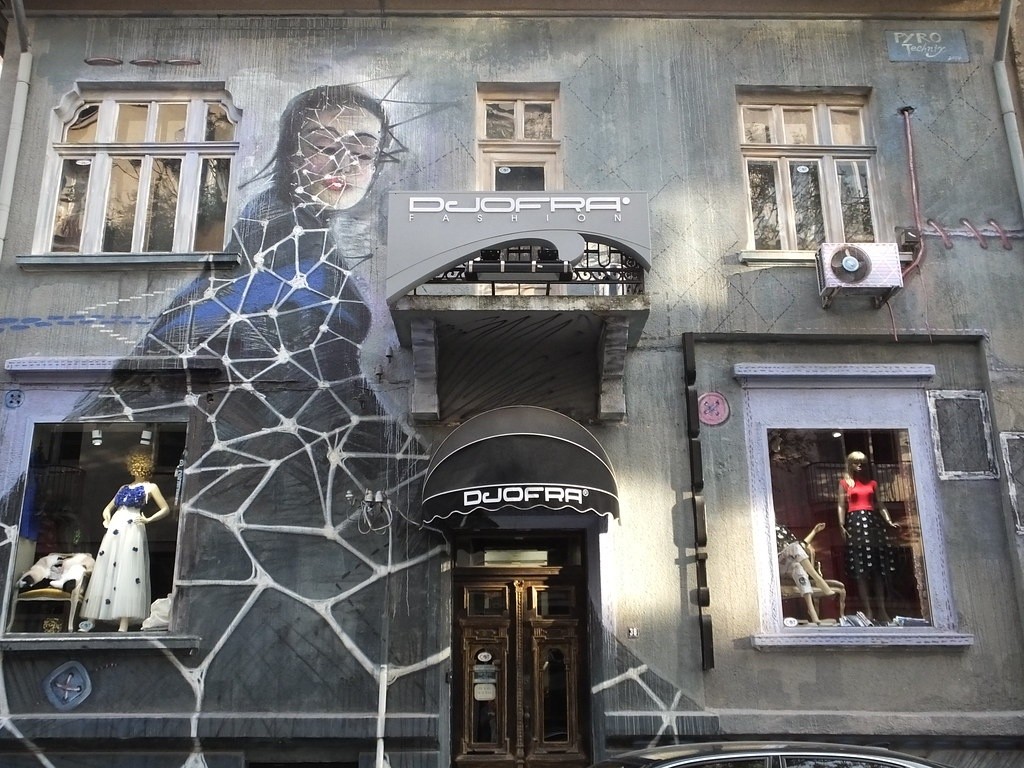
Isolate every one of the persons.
[837,451,901,623]
[776,522,835,625]
[80,448,170,633]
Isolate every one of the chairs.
[6,551,95,632]
[778,536,847,622]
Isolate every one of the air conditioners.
[814,243,904,309]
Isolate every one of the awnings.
[418,404,621,532]
